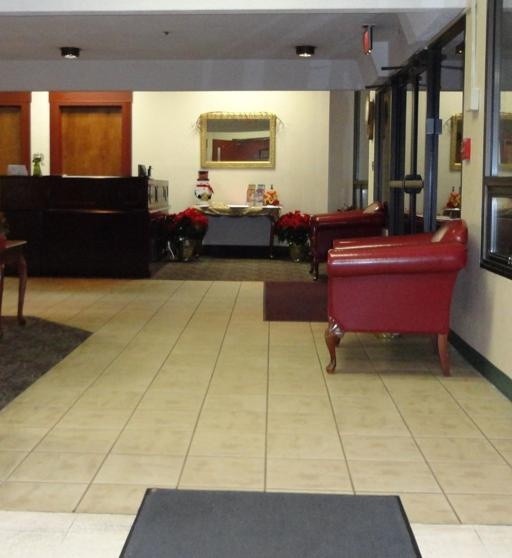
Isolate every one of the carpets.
[263,277,330,323]
[0,313,93,412]
[117,486,421,557]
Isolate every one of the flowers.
[165,207,209,244]
[271,209,315,243]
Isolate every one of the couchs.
[324,219,469,377]
[310,202,384,281]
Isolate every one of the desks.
[0,239,28,327]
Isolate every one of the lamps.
[294,45,316,58]
[60,47,80,59]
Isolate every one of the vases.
[178,238,198,260]
[288,241,307,262]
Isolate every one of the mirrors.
[199,113,276,170]
[450,115,461,170]
